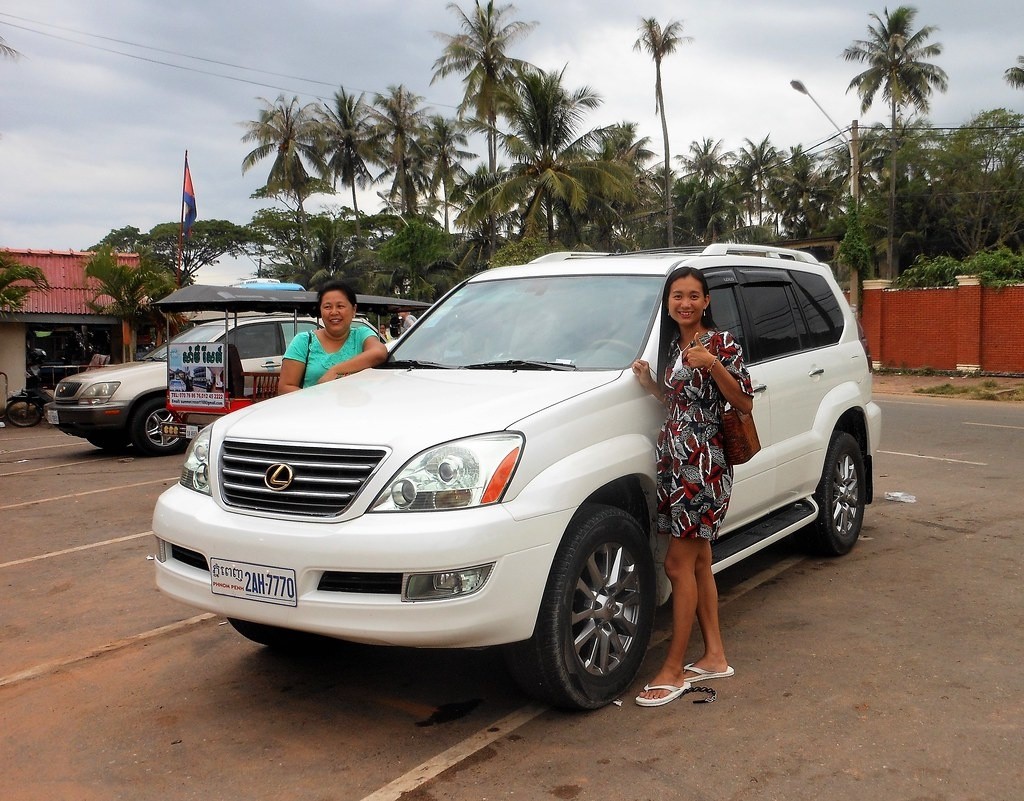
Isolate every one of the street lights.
[788,78,859,321]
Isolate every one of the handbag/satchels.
[709,330,762,465]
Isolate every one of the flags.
[183,160,198,239]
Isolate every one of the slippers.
[635,682,692,707]
[683,662,735,683]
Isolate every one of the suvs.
[43,315,389,457]
[150,242,887,711]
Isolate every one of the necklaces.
[682,343,690,351]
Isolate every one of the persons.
[398,310,417,329]
[630,266,755,706]
[380,324,391,341]
[276,280,387,395]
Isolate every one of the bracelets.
[706,356,718,373]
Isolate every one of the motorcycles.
[5,341,57,429]
